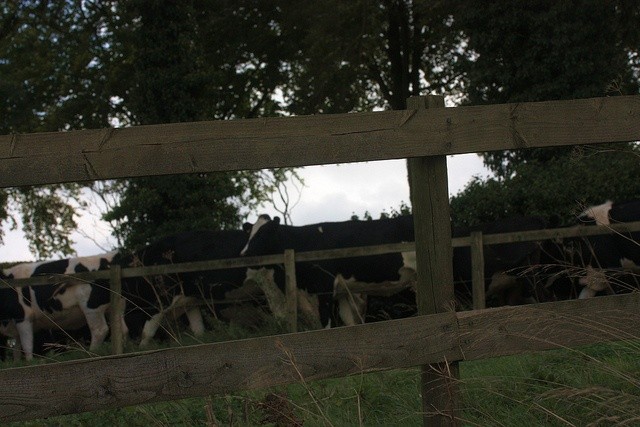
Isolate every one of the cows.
[0,250,134,363]
[113,231,254,352]
[239,214,421,328]
[564,199,640,301]
[450,214,567,310]
